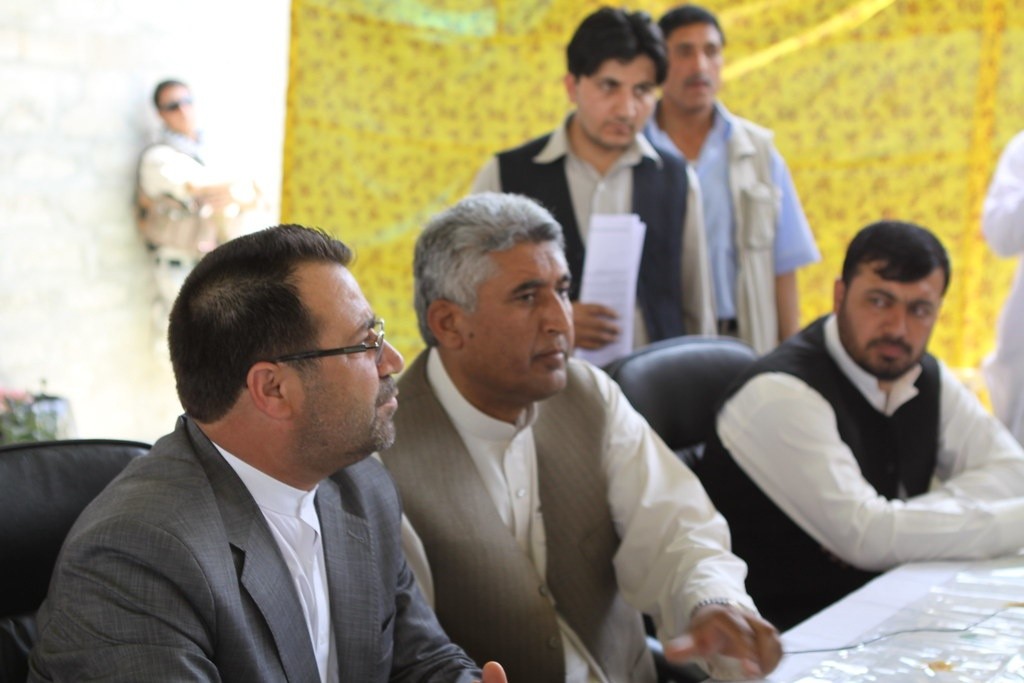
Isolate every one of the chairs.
[0,438,154,683]
[600,333,761,683]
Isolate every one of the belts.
[155,260,182,267]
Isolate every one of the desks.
[702,544,1024,683]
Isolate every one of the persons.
[136,6,1024,632]
[365,192,786,683]
[28,223,512,683]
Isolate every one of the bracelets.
[691,599,749,620]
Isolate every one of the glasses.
[164,97,194,111]
[244,319,385,389]
